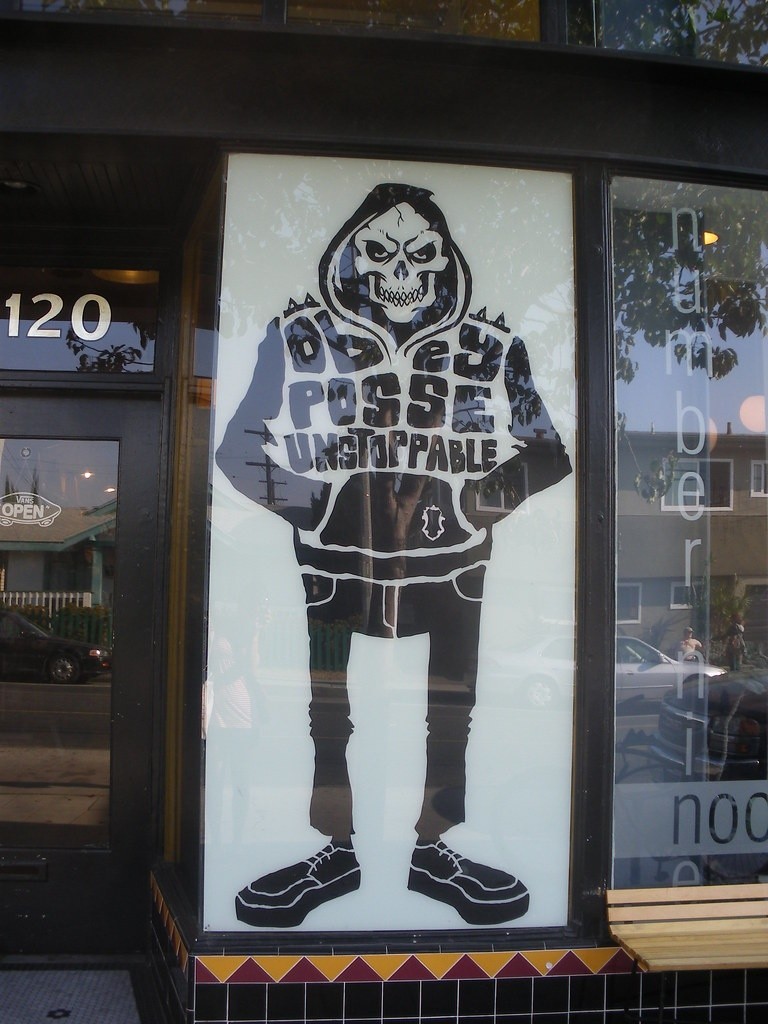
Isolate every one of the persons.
[723,615,746,670]
[675,627,703,662]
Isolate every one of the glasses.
[687,631,692,634]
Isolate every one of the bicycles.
[616,727,749,885]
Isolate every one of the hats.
[684,627,693,632]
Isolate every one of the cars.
[527,636,727,712]
[0,609,108,685]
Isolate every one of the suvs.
[659,667,768,885]
